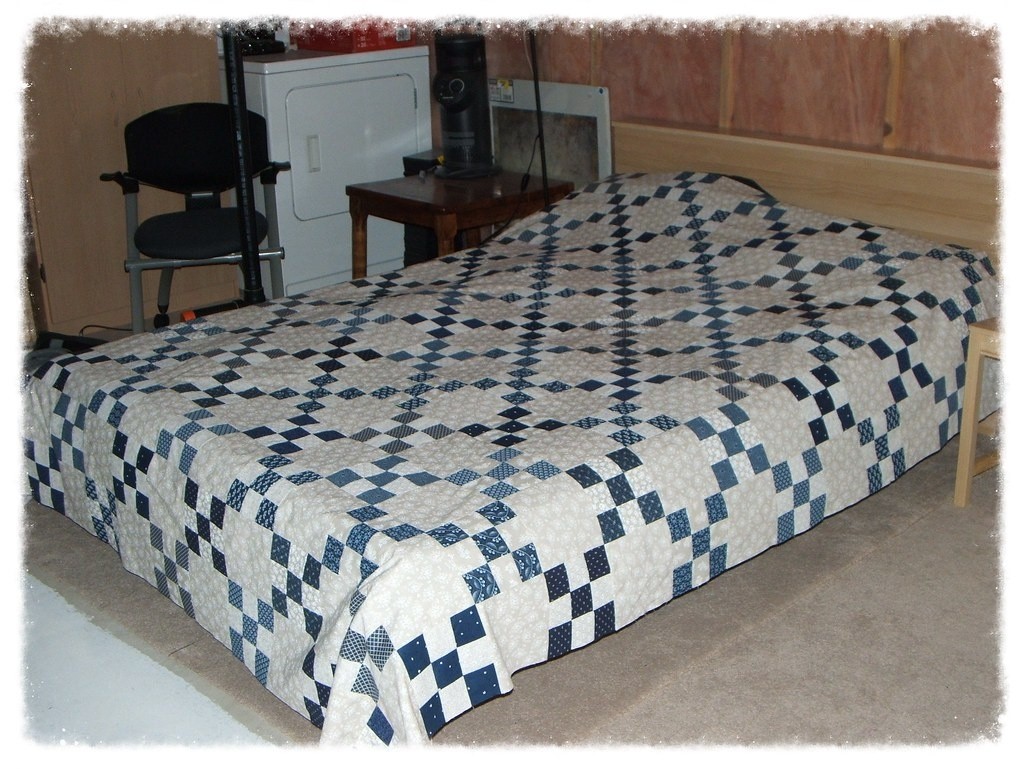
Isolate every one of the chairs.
[99,101,291,335]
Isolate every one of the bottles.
[180,298,242,322]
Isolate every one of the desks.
[345,170,574,280]
[955,318,1000,507]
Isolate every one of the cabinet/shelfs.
[241,46,438,300]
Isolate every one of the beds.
[22,122,1000,746]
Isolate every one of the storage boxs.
[289,20,416,53]
